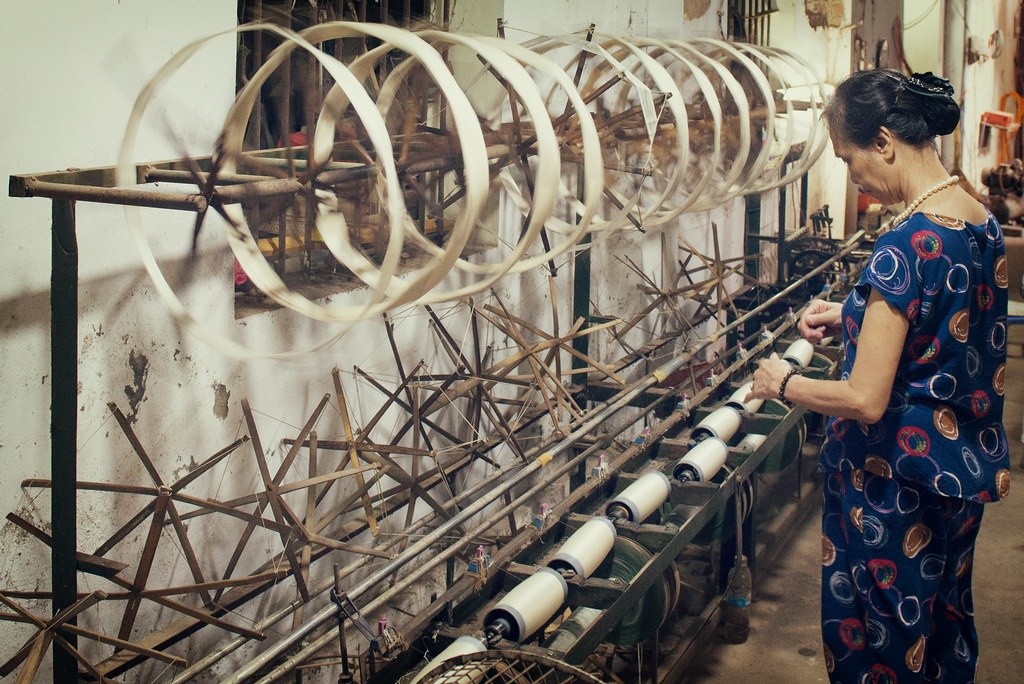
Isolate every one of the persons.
[741,68,1009,683]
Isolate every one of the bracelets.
[779,369,805,407]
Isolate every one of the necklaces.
[893,175,959,227]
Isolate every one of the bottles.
[723,554,752,641]
